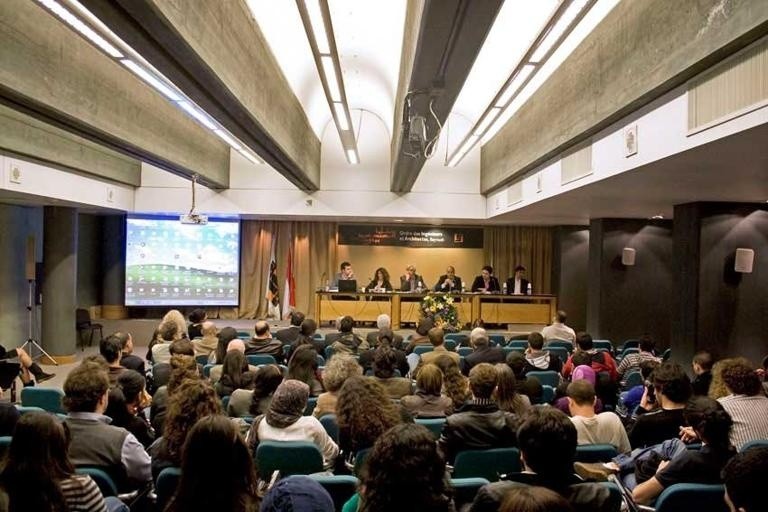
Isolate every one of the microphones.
[320,272,327,286]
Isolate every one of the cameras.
[643,380,656,404]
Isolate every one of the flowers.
[419,290,465,332]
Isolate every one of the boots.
[23,380,34,387]
[28,363,56,384]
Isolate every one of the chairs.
[414,418,447,441]
[413,345,434,355]
[394,369,402,379]
[622,349,639,361]
[574,444,617,465]
[444,340,456,351]
[592,340,611,350]
[546,340,573,355]
[196,354,209,365]
[325,346,331,359]
[526,370,559,387]
[445,334,470,346]
[243,416,254,424]
[740,440,768,453]
[18,406,43,419]
[597,347,609,353]
[503,347,525,356]
[1,436,13,456]
[625,372,641,388]
[509,340,529,349]
[221,396,230,410]
[75,466,117,500]
[456,347,473,356]
[303,398,318,416]
[156,468,181,504]
[271,332,277,337]
[489,335,508,346]
[620,340,640,354]
[634,482,726,512]
[312,474,360,512]
[76,308,103,354]
[541,383,553,405]
[318,414,338,443]
[282,345,291,355]
[455,448,523,480]
[236,331,250,336]
[203,364,215,378]
[318,355,325,366]
[314,333,321,338]
[247,354,278,367]
[257,441,323,485]
[20,386,66,413]
[447,476,490,510]
[543,347,567,361]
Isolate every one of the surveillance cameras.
[23,230,36,280]
[408,116,427,152]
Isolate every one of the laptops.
[338,280,356,293]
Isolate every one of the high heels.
[362,321,366,326]
[370,322,377,327]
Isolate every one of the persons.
[154,337,203,388]
[626,362,692,445]
[618,358,659,413]
[434,266,462,292]
[1,411,133,512]
[80,352,115,388]
[341,448,375,512]
[266,260,282,321]
[191,321,219,359]
[557,351,601,394]
[541,310,576,354]
[288,319,325,364]
[499,485,571,512]
[188,308,214,340]
[401,364,453,417]
[260,475,334,512]
[146,380,250,466]
[100,335,127,390]
[521,332,558,372]
[720,446,768,512]
[277,311,306,345]
[561,331,618,385]
[505,351,528,393]
[209,339,259,382]
[691,352,714,395]
[0,345,56,392]
[59,361,157,503]
[425,354,473,409]
[357,422,457,512]
[332,316,370,357]
[708,357,751,399]
[438,363,522,467]
[573,395,735,506]
[212,350,256,400]
[755,355,768,399]
[227,364,284,418]
[367,316,404,349]
[112,330,146,379]
[326,316,349,345]
[152,355,209,407]
[207,327,238,363]
[148,309,189,348]
[311,351,364,419]
[335,375,402,442]
[472,266,500,293]
[616,335,663,385]
[400,265,428,291]
[522,376,552,408]
[163,413,264,512]
[565,379,632,457]
[468,407,585,512]
[152,320,190,364]
[245,379,340,469]
[494,363,532,429]
[715,364,768,454]
[245,321,283,363]
[507,265,532,294]
[553,365,603,417]
[325,332,363,358]
[365,268,393,291]
[283,344,324,397]
[464,327,504,372]
[411,317,435,343]
[420,327,460,371]
[359,327,398,369]
[103,369,156,449]
[335,262,359,291]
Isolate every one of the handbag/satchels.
[633,438,688,485]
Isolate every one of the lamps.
[734,248,756,273]
[621,247,636,266]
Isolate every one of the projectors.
[180,215,208,225]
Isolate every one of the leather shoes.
[491,323,499,329]
[401,322,410,328]
[414,324,417,328]
[483,323,491,329]
[498,323,507,329]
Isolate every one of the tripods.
[20,280,59,366]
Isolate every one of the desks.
[314,289,558,333]
